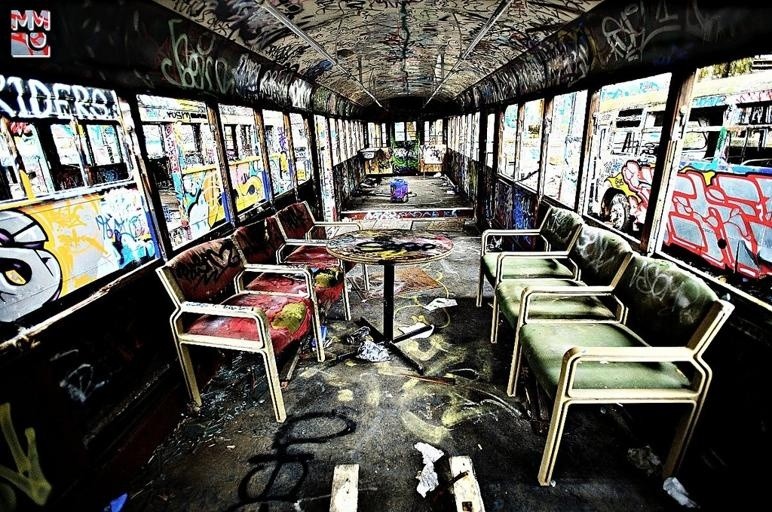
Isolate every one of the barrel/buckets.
[390,178,409,203]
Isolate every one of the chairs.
[154,200,370,424]
[476,206,736,487]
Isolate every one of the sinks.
[360,147,380,159]
